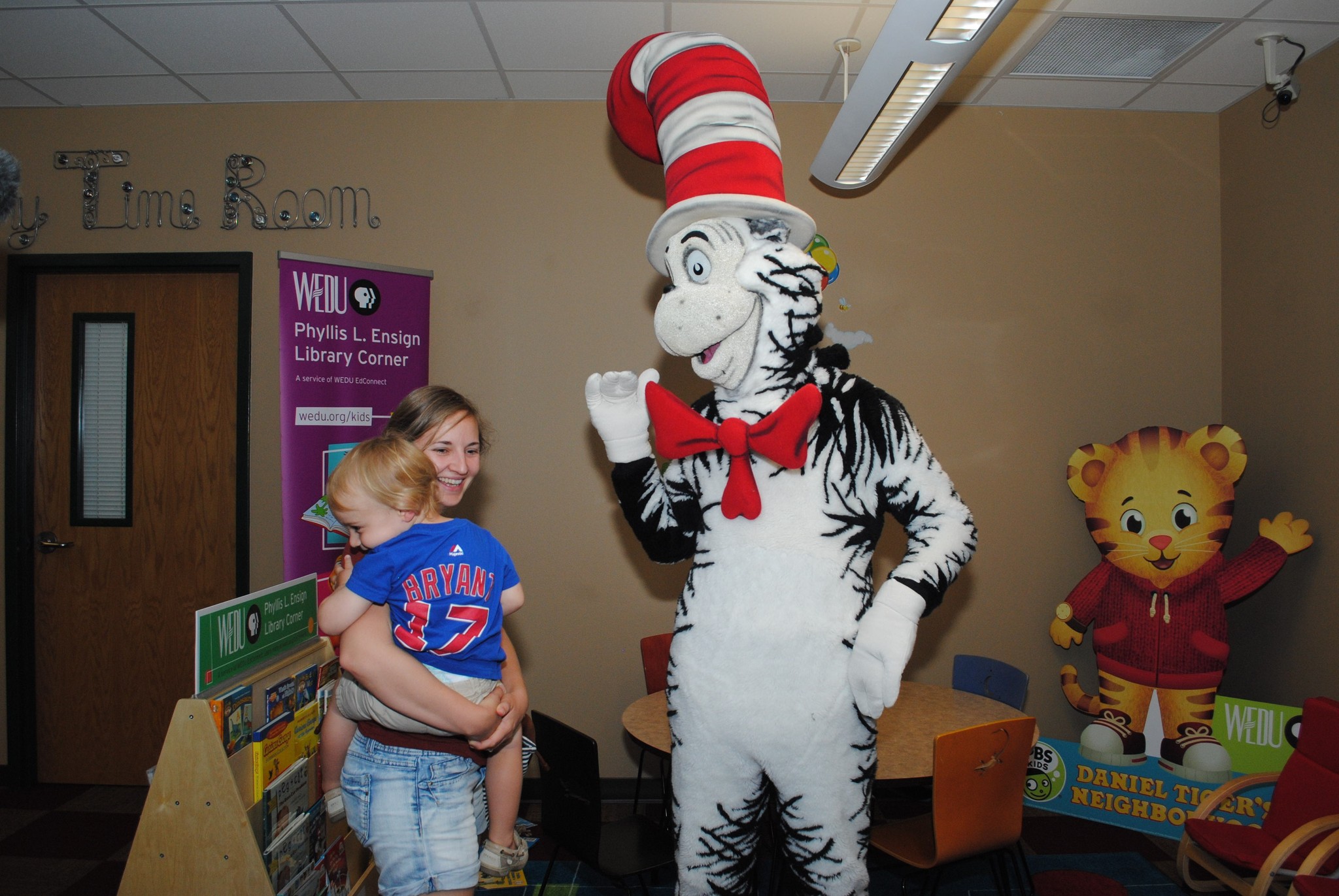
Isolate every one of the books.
[210,655,374,896]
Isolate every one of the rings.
[488,749,493,754]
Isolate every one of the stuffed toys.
[585,30,979,896]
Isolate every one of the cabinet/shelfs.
[115,635,382,896]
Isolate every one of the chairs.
[951,652,1030,712]
[630,631,676,832]
[1173,695,1339,896]
[529,707,676,896]
[864,717,1039,896]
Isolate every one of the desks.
[620,679,1041,885]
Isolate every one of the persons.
[318,384,530,896]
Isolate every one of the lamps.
[807,0,1021,191]
[1254,31,1305,121]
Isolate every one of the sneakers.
[480,828,530,876]
[323,788,347,824]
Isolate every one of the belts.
[357,719,486,765]
